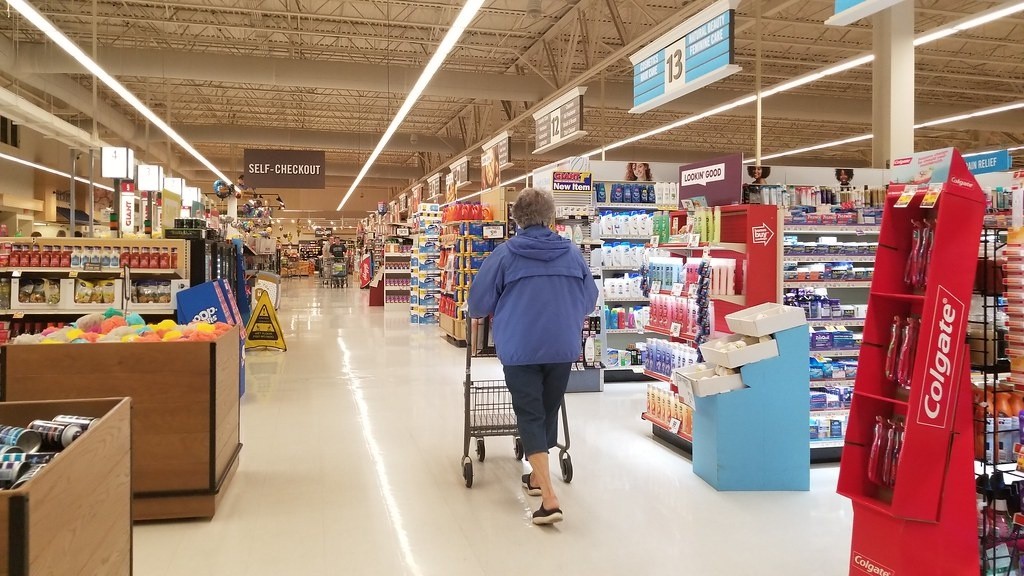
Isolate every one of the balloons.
[215,175,293,248]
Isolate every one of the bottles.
[0,415,100,490]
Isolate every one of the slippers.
[521,473,542,495]
[532,501,563,524]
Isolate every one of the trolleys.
[323,256,348,289]
[462,310,574,488]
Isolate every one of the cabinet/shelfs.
[836,291,929,515]
[598,201,673,372]
[777,223,881,451]
[970,228,1024,576]
[384,223,410,303]
[442,233,507,319]
[0,261,194,347]
[641,242,743,442]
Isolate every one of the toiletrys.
[643,211,735,438]
[739,183,886,207]
[0,243,178,269]
[971,381,1024,576]
[980,186,1014,208]
[558,181,680,365]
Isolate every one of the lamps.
[5,0,272,220]
[100,147,201,207]
[337,0,1024,213]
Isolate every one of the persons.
[625,163,653,181]
[317,237,335,284]
[329,237,347,288]
[745,166,771,184]
[470,189,598,524]
[836,169,854,185]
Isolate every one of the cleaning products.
[438,201,506,322]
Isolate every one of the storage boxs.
[0,323,242,521]
[0,397,131,576]
[9,267,190,311]
[410,202,442,325]
[676,302,805,395]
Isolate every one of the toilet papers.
[409,203,443,327]
